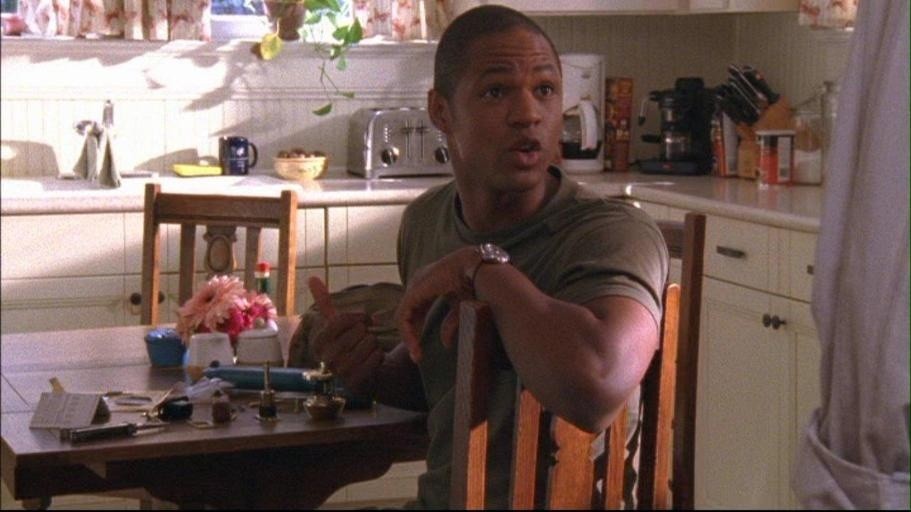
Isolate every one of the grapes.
[278,148,323,159]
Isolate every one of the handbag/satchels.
[286,281,432,412]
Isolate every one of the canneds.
[756,127,797,187]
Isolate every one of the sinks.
[1,170,307,201]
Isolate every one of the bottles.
[792,79,840,186]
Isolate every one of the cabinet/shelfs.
[694,206,822,510]
[0,208,323,319]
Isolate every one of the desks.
[3,328,432,512]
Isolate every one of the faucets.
[74,99,113,134]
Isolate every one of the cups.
[2,12,26,36]
[219,135,258,175]
[144,327,185,368]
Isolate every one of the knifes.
[709,60,781,125]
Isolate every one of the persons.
[302,2,675,509]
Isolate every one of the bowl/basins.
[272,155,327,181]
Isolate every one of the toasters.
[343,102,455,180]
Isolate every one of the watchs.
[465,243,510,288]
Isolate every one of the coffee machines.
[556,51,608,177]
[626,76,719,177]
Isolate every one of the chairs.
[451,284,679,512]
[142,183,295,325]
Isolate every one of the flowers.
[174,273,278,344]
